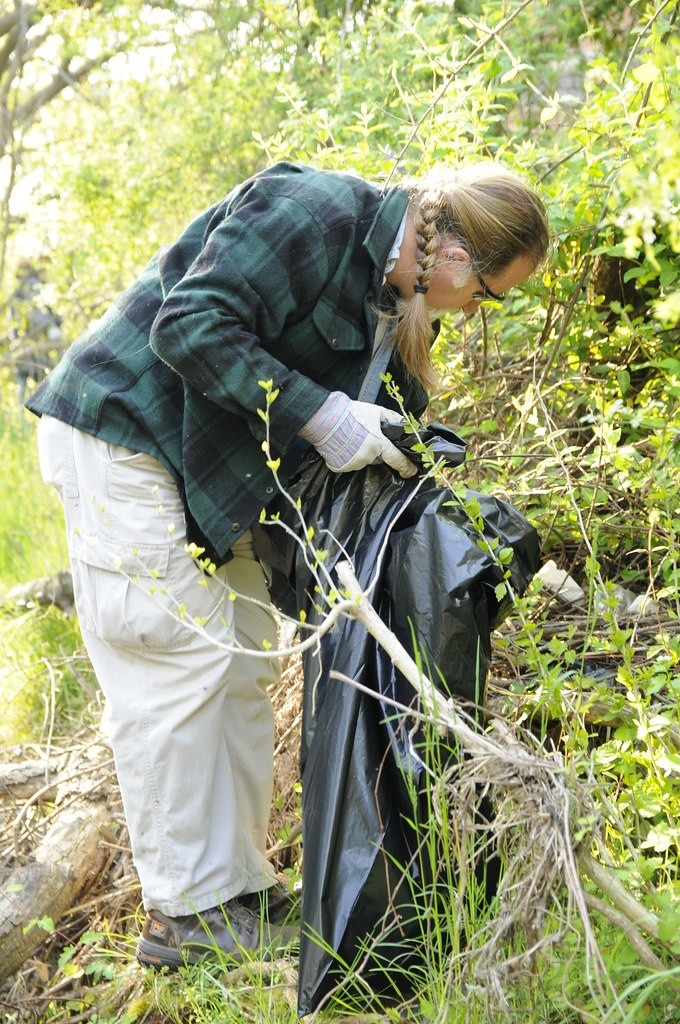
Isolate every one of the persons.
[24,162,550,985]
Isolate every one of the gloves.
[298,391,420,479]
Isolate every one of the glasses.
[461,244,507,304]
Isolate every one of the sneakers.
[136,900,302,976]
[240,883,299,923]
